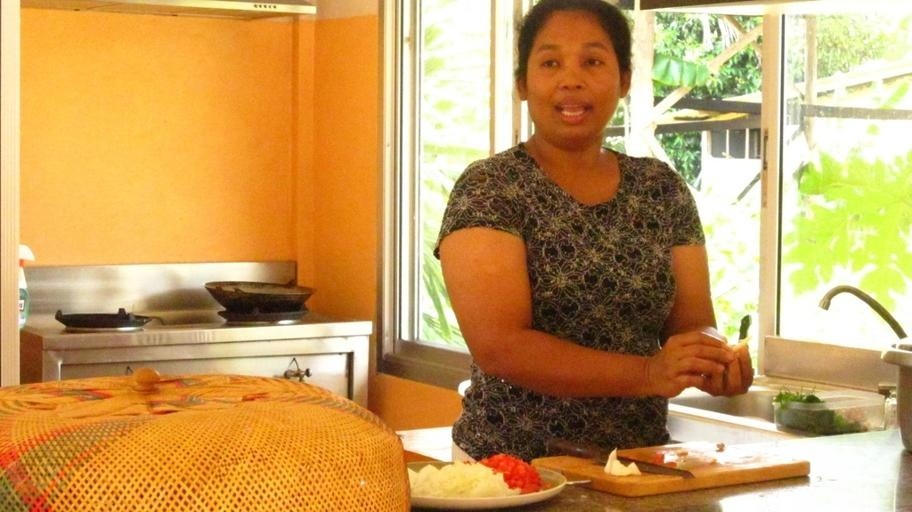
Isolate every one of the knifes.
[543,434,694,482]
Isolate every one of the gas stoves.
[20,303,374,410]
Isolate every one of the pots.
[197,277,318,313]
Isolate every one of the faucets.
[818,284,906,431]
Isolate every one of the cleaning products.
[18,244,34,327]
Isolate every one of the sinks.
[665,383,896,439]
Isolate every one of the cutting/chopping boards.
[529,441,814,498]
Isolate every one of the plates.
[406,457,568,512]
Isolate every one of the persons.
[432,0,755,464]
